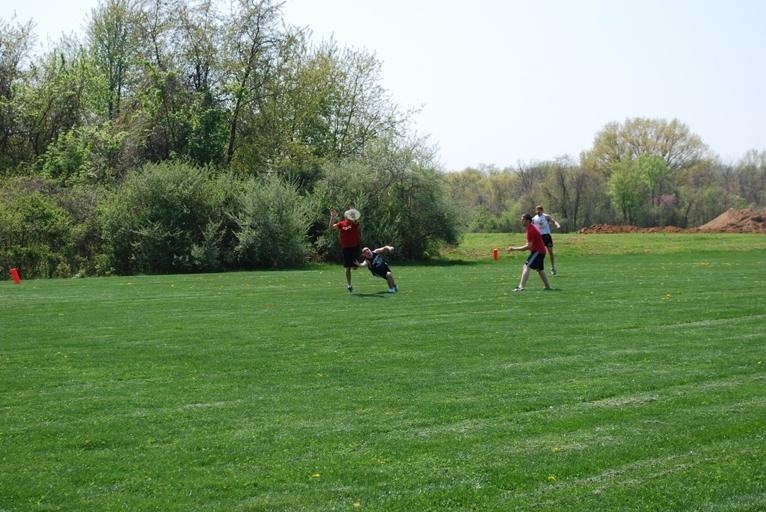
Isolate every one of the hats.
[345,208,361,221]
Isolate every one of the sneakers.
[388,286,398,293]
[551,268,556,275]
[512,286,524,291]
[348,286,353,294]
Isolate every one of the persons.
[353,246,398,293]
[505,213,552,292]
[328,207,362,295]
[531,205,561,275]
